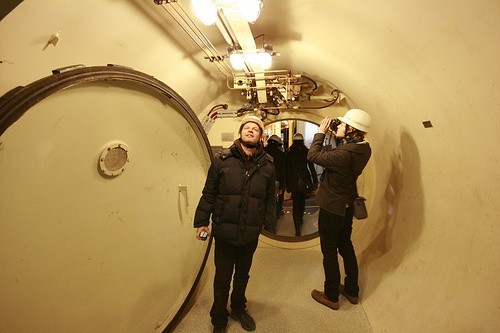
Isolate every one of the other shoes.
[231,309,256,331]
[311,289,339,310]
[213,325,226,333]
[340,284,358,304]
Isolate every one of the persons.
[192,116,278,333]
[264,133,318,236]
[306,108,372,310]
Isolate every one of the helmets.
[292,133,304,141]
[337,109,371,133]
[278,137,284,144]
[238,116,264,136]
[270,135,279,142]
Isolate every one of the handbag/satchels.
[352,196,368,220]
[298,171,315,194]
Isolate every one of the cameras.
[329,119,342,132]
[199,231,208,241]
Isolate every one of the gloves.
[312,182,319,191]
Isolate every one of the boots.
[294,215,303,236]
